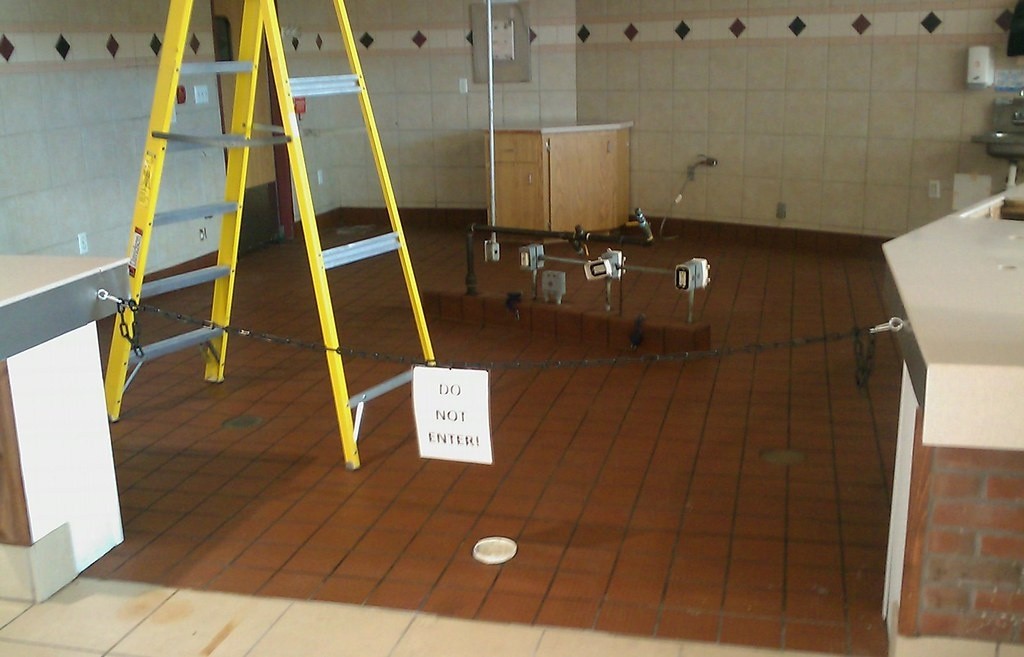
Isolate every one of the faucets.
[1011,111,1024,125]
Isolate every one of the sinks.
[970,132,1024,160]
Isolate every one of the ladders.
[105,0,437,471]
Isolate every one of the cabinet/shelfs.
[484,119,634,244]
